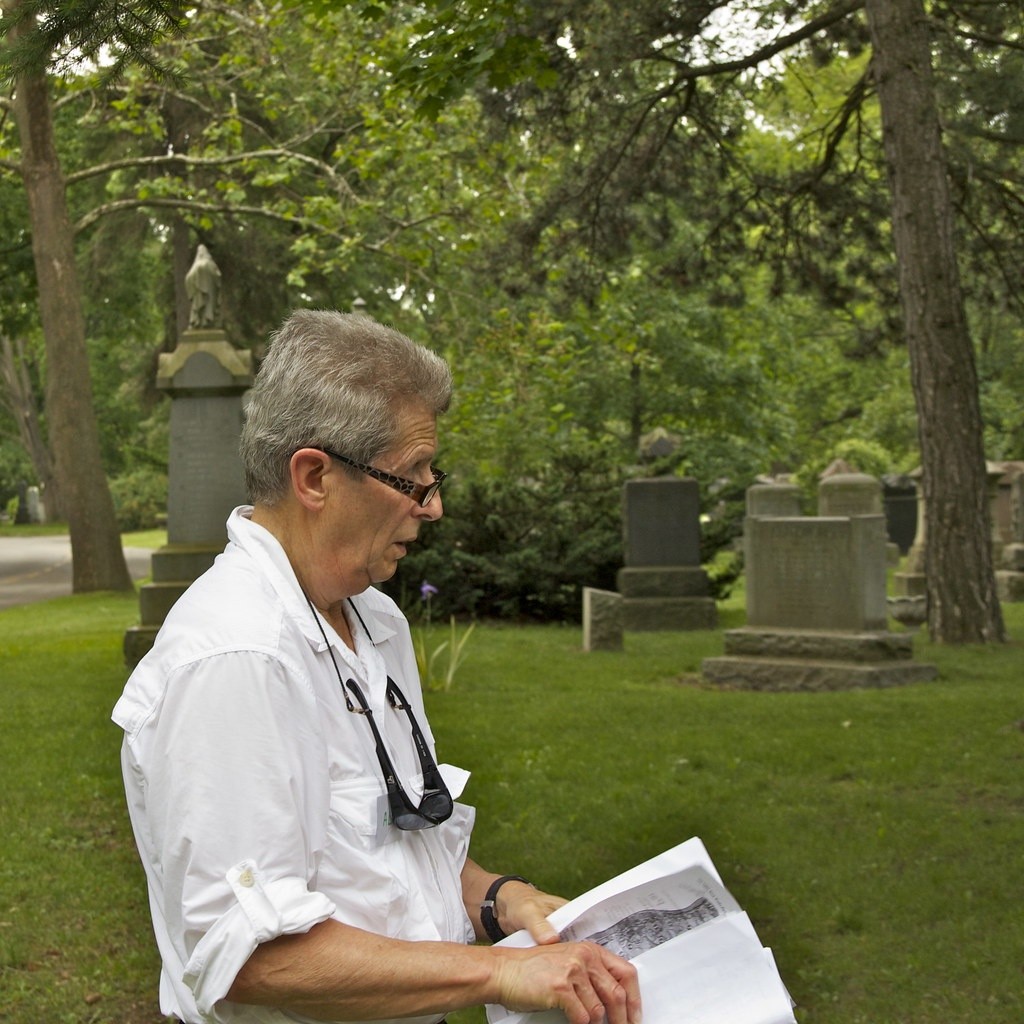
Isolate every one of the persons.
[111,310,641,1024]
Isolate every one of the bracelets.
[480,875,535,943]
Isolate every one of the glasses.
[296,443,449,509]
[346,675,455,832]
